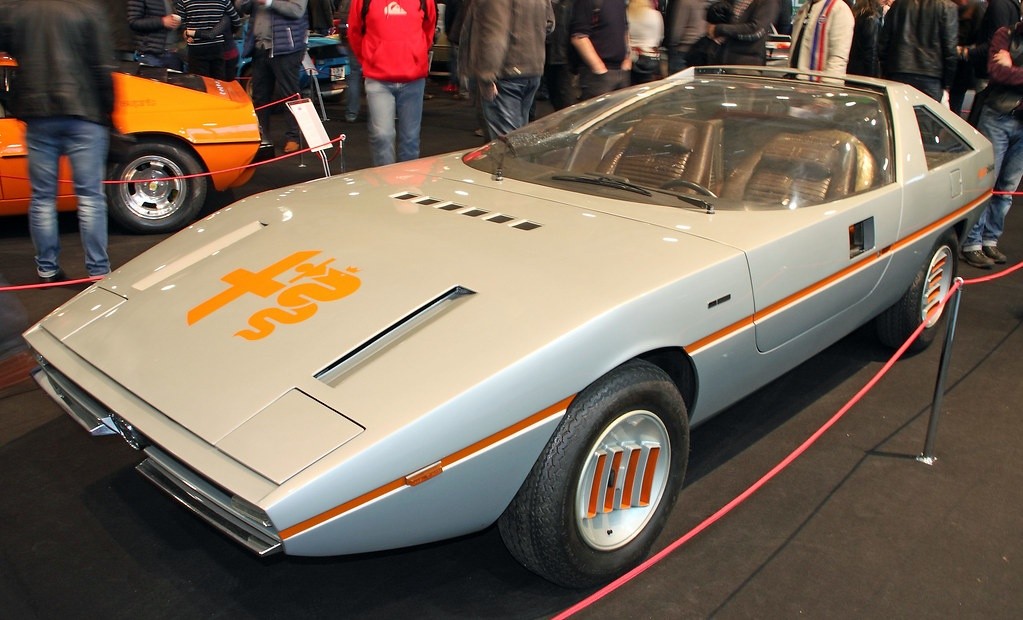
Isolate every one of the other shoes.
[345,113,357,123]
[424,93,432,99]
[88,268,112,283]
[454,90,470,100]
[475,129,489,137]
[37,265,60,278]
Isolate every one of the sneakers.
[959,249,995,268]
[983,245,1008,264]
[285,140,300,153]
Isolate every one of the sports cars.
[220,23,353,106]
[19,63,996,591]
[0,50,262,235]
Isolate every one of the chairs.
[723,129,874,208]
[597,113,714,197]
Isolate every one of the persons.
[234,0,310,153]
[346,0,436,166]
[423,0,470,100]
[460,0,580,137]
[2,0,114,290]
[307,0,365,121]
[125,0,243,84]
[950,0,1023,112]
[474,0,556,144]
[568,0,633,103]
[626,0,1022,144]
[787,98,836,123]
[958,0,1023,268]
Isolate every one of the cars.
[425,2,462,78]
[760,0,820,79]
